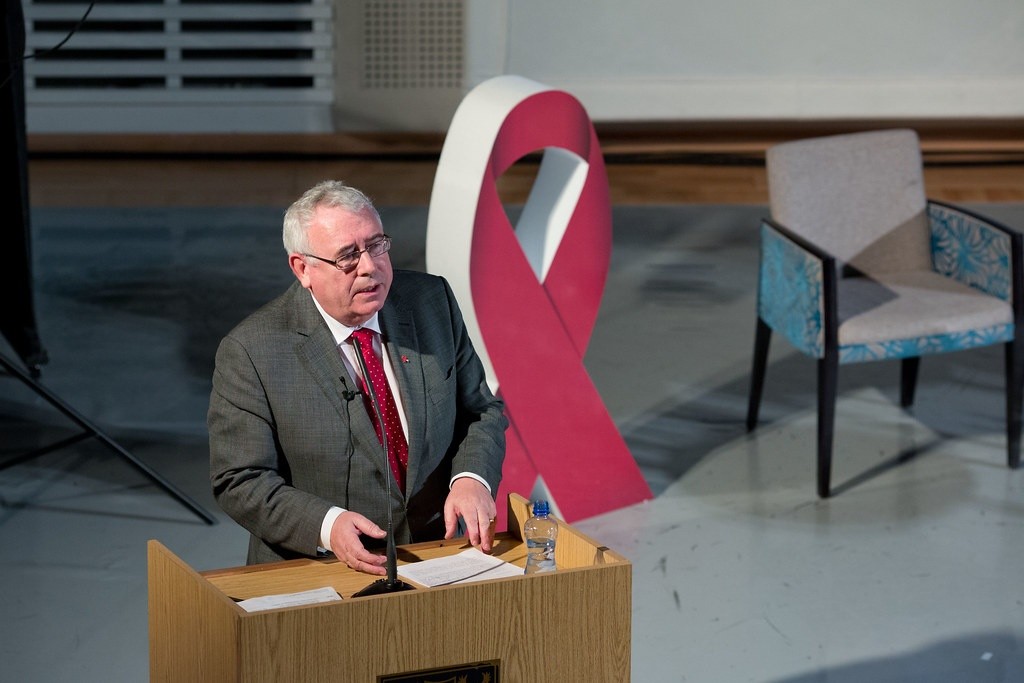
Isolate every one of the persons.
[206,180,510,575]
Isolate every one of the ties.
[350,328,409,502]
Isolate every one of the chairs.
[745,130,1024,501]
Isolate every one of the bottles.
[524,499,557,574]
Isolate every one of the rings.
[489,519,494,522]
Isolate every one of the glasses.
[299,235,393,270]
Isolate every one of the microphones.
[352,338,417,600]
[338,377,346,385]
